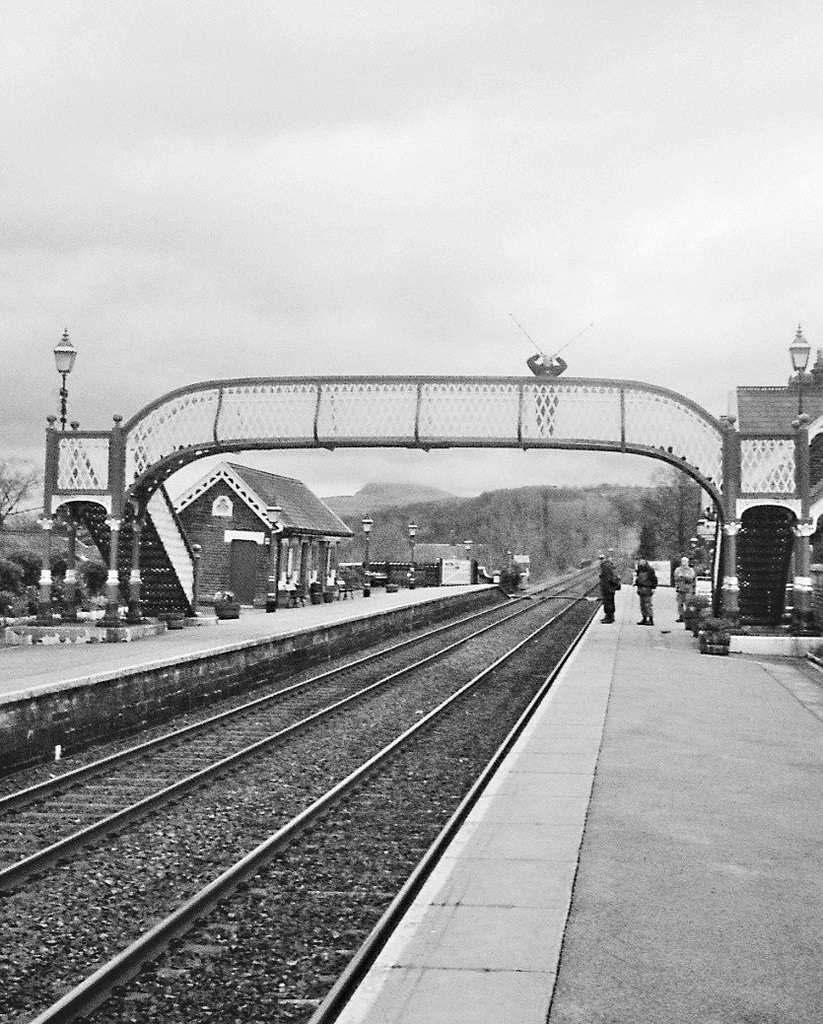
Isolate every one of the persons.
[526,352,568,438]
[598,554,622,625]
[672,556,696,623]
[632,556,658,626]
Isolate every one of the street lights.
[464,536,473,561]
[789,323,812,415]
[408,519,417,589]
[266,494,281,613]
[54,328,78,430]
[361,512,373,597]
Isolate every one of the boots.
[602,613,615,623]
[638,617,648,625]
[645,617,653,625]
